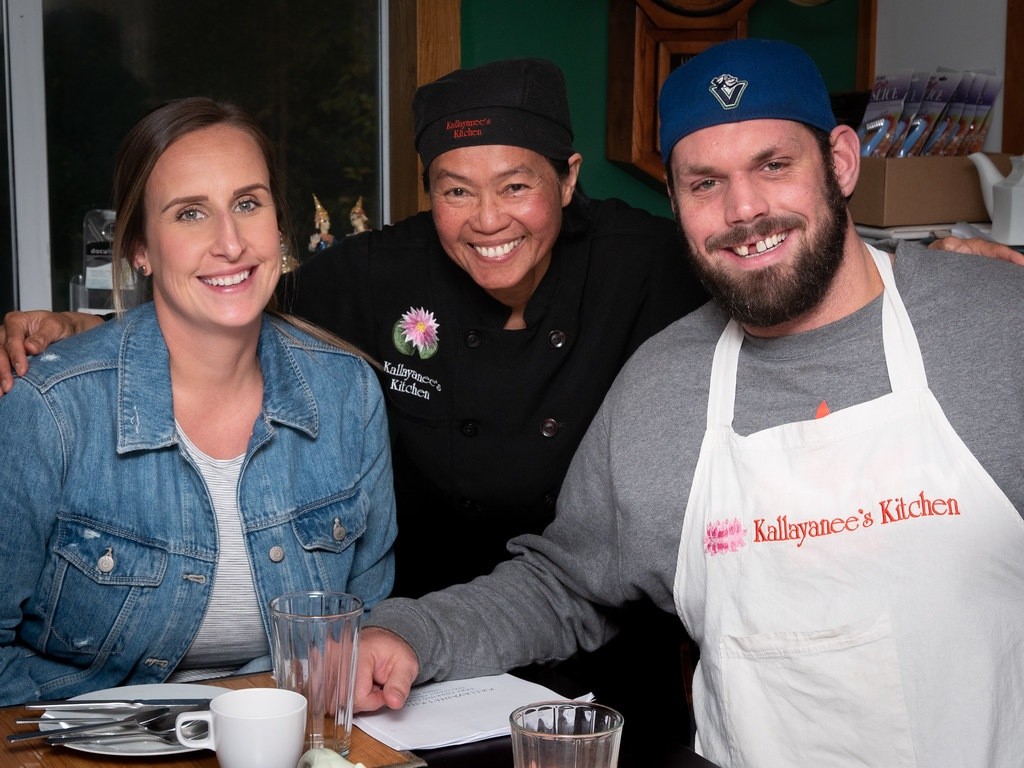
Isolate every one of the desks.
[412,670,718,768]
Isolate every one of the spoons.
[6,711,196,744]
[46,722,208,747]
[15,708,171,728]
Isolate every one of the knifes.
[24,698,212,711]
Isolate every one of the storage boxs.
[846,151,1015,228]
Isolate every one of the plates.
[38,683,233,756]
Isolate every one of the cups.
[267,591,365,759]
[509,700,624,768]
[175,688,307,768]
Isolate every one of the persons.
[0,38,1024,768]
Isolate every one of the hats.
[410,56,575,170]
[659,38,836,168]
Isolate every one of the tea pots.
[966,152,1024,248]
